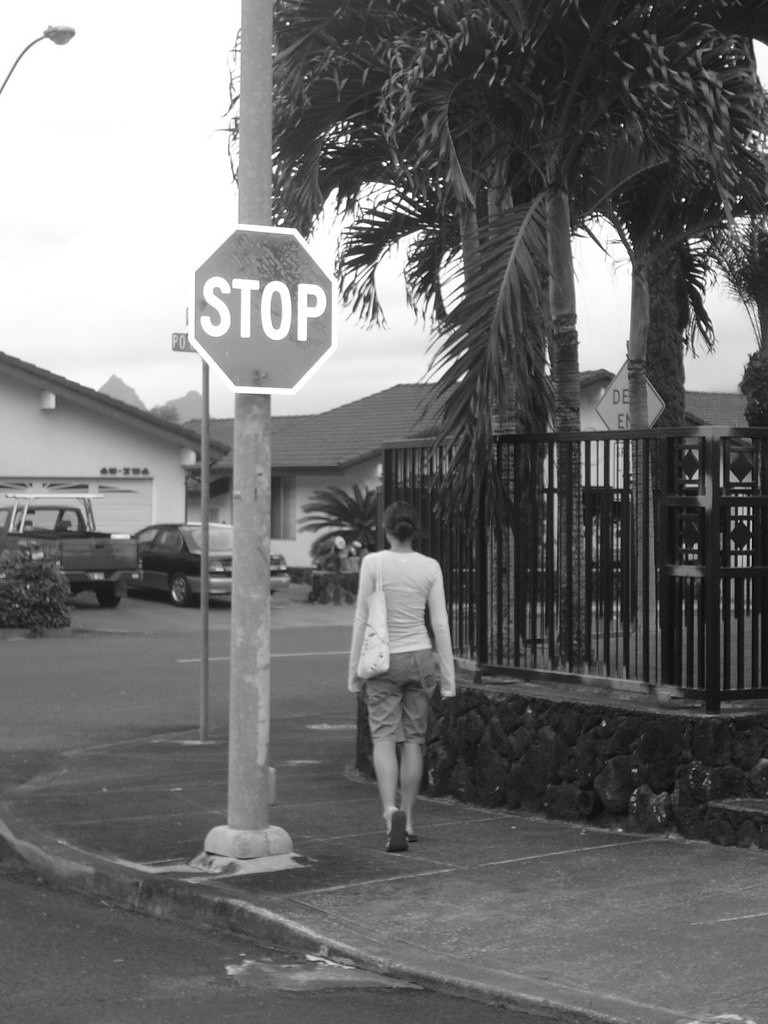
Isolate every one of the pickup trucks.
[0,491,144,609]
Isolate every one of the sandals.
[382,807,418,852]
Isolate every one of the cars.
[120,521,290,606]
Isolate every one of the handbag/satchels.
[355,550,390,680]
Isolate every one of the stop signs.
[190,224,333,395]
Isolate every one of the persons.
[348,501,456,852]
